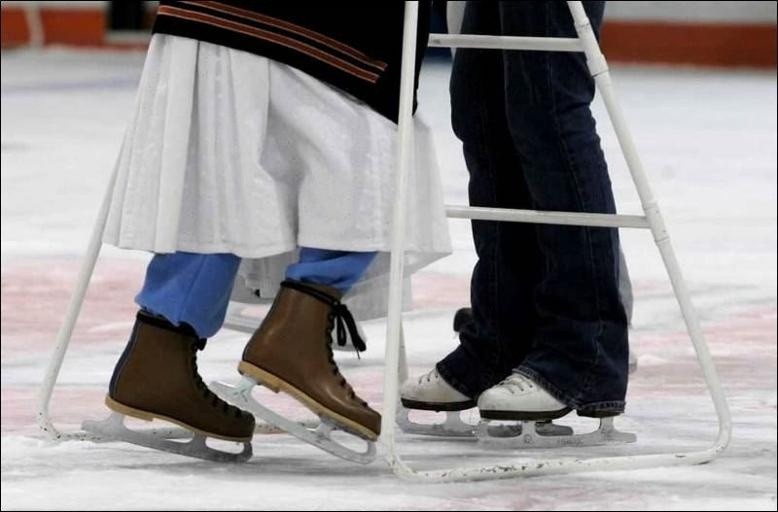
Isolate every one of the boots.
[104,309,256,444]
[238,281,382,441]
[399,345,628,418]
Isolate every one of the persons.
[102,1,453,438]
[398,1,629,417]
